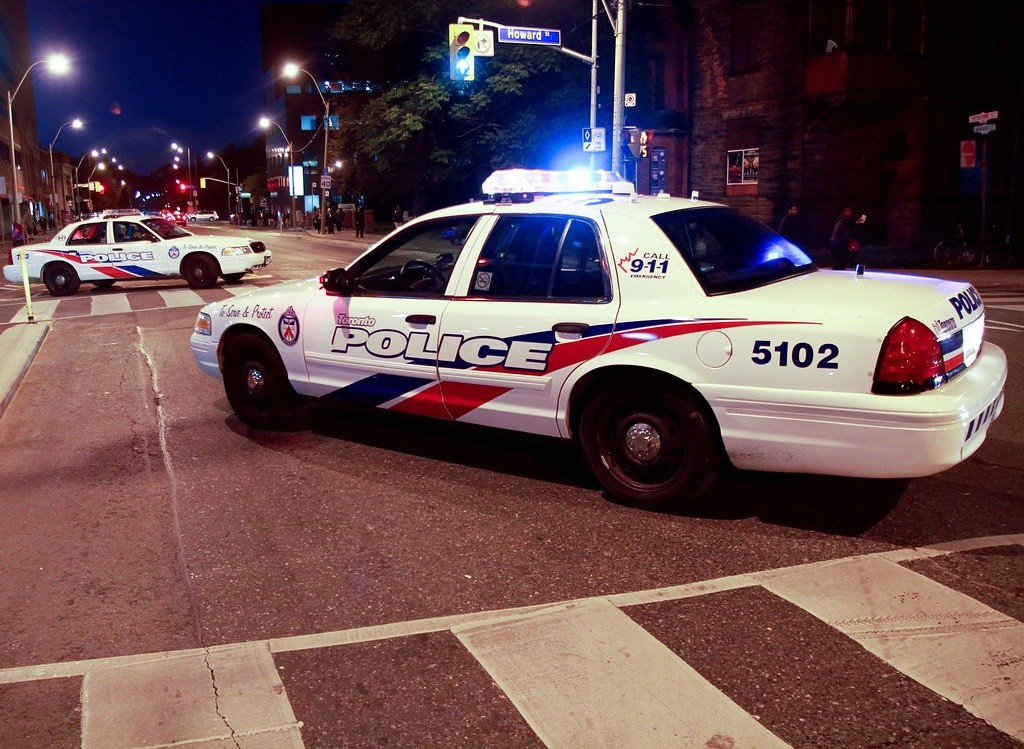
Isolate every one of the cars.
[191,170,1008,509]
[2,207,271,300]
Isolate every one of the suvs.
[189,210,219,223]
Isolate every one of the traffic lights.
[449,24,474,81]
[640,131,654,158]
[621,129,640,159]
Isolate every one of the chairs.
[123,226,136,241]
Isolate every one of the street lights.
[170,142,198,211]
[207,150,232,225]
[7,50,76,246]
[257,115,297,228]
[518,0,598,170]
[69,148,126,220]
[280,61,330,235]
[48,118,87,235]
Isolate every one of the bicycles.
[934,223,1013,272]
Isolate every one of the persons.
[354,206,365,238]
[313,206,345,234]
[12,215,53,248]
[133,224,172,242]
[829,207,864,270]
[393,206,401,230]
[243,209,290,228]
[778,206,799,244]
